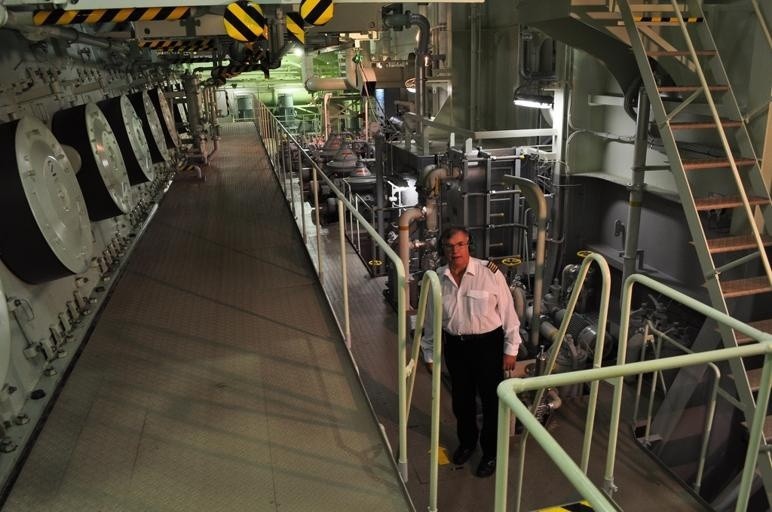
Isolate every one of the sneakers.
[452,444,477,465]
[472,460,496,478]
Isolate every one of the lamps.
[512,84,553,109]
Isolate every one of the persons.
[423,227,523,478]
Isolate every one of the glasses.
[443,241,467,251]
